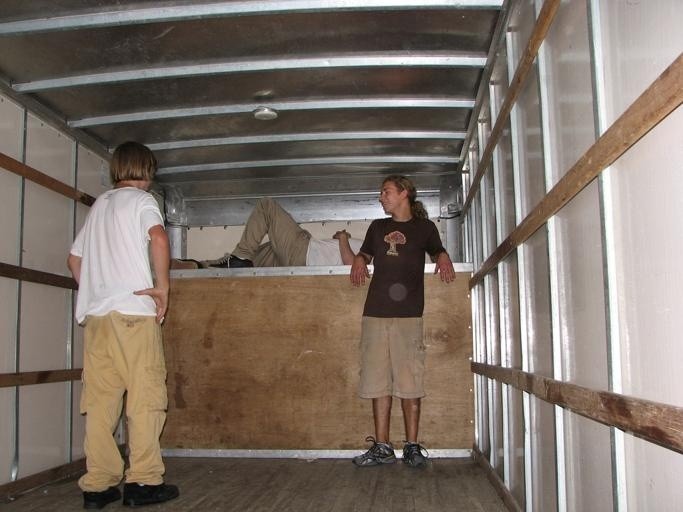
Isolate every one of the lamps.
[254,108,279,120]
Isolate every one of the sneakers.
[124,482,179,505]
[208,254,253,268]
[169,258,203,269]
[401,443,426,469]
[83,487,121,508]
[353,440,397,466]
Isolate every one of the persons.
[349,174,457,468]
[169,196,375,270]
[67,141,179,510]
